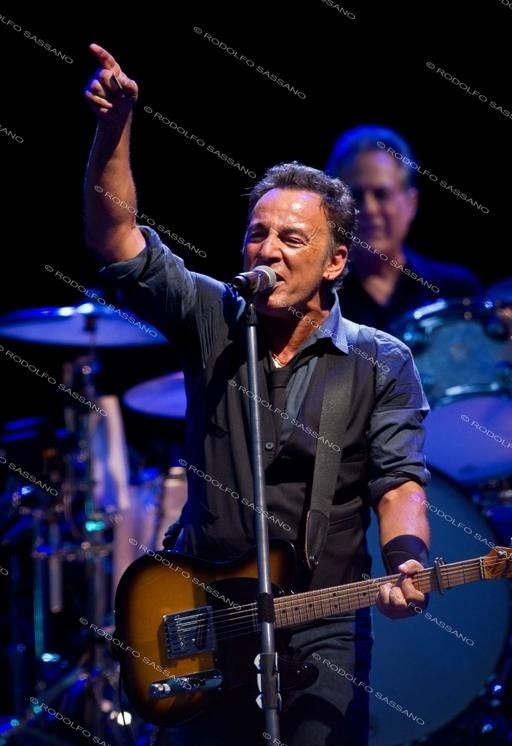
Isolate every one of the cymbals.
[1,303,167,347]
[126,372,190,418]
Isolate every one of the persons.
[326,123,483,333]
[80,40,434,746]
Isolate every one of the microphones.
[230,265,276,295]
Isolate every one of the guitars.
[114,537,512,728]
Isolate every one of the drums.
[365,465,511,745]
[388,299,511,491]
